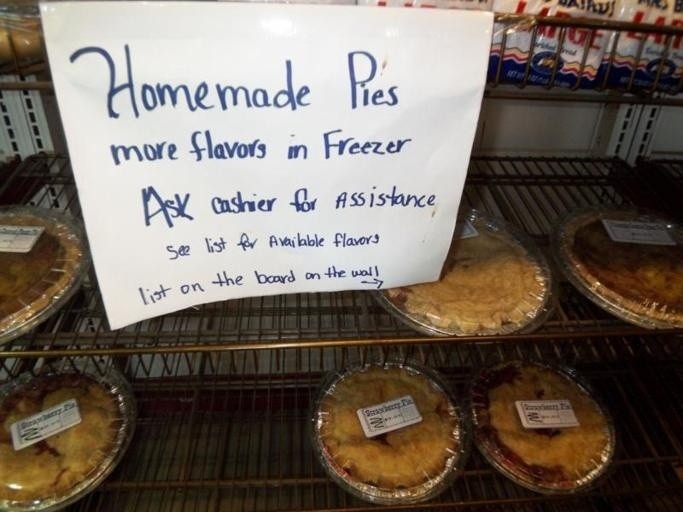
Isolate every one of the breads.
[0,19,46,67]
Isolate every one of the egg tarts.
[468,357,618,494]
[0,205,87,340]
[1,362,126,511]
[308,359,467,505]
[388,213,552,335]
[553,207,683,333]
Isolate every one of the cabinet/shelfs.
[1,8,682,511]
